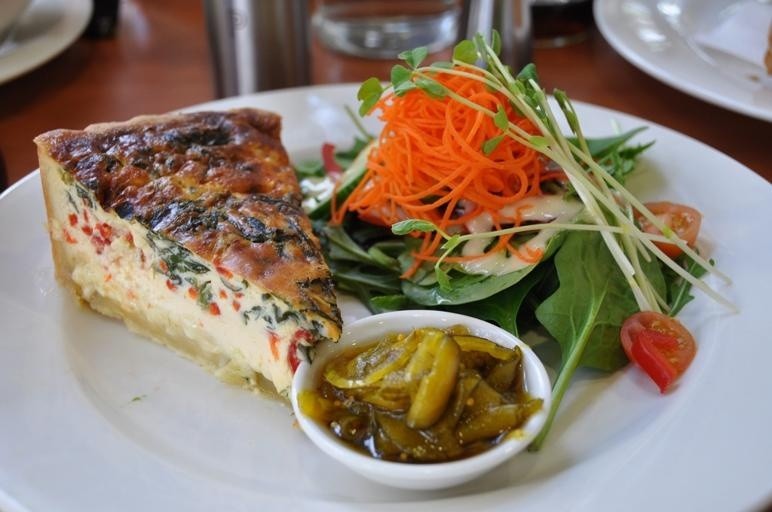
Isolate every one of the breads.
[34,107,342,406]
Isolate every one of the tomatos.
[620,311,696,394]
[634,202,701,258]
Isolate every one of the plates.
[0,1,772,511]
